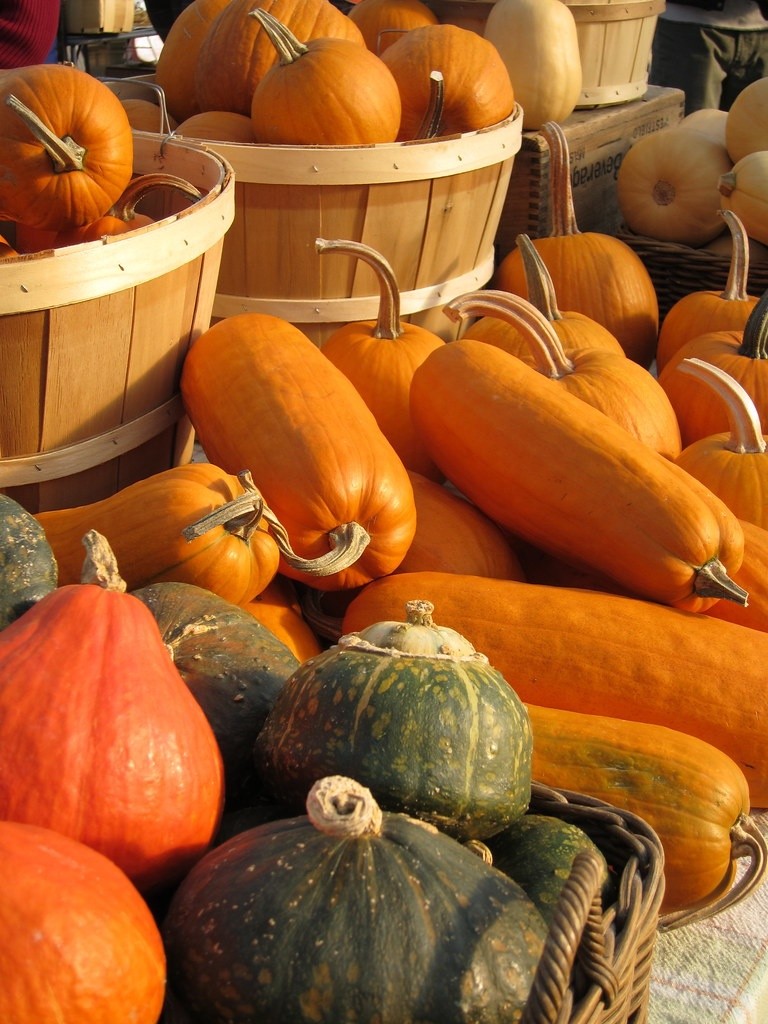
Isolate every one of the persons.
[0,0,64,69]
[648,0,768,119]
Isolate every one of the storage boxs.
[494,85,687,264]
[63,0,136,35]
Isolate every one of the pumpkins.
[0,0,768,1024]
